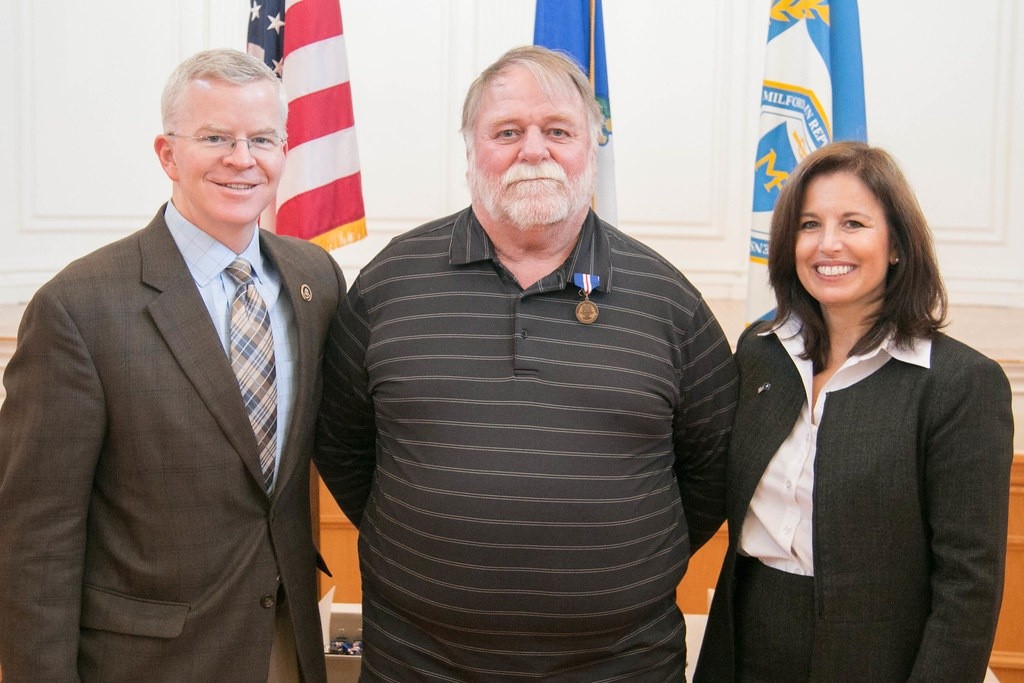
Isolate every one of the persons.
[692,141,1015,683]
[311,46,739,683]
[0,48,346,683]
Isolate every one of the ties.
[228,258,276,496]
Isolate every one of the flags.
[534,0,618,229]
[247,0,367,253]
[745,0,867,330]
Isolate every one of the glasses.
[169,133,287,151]
